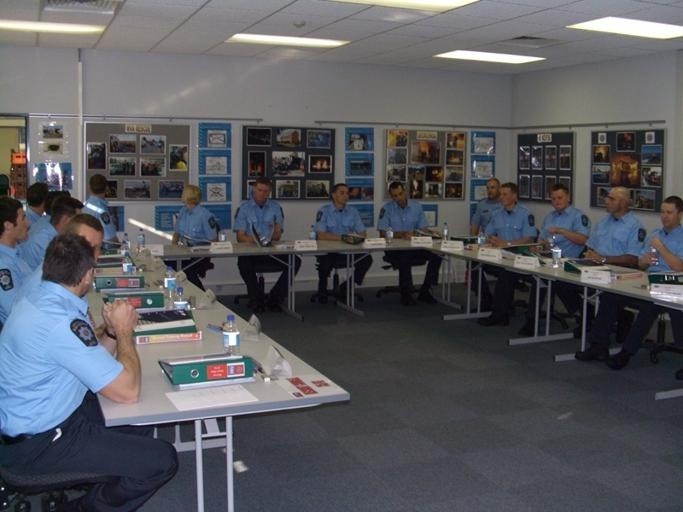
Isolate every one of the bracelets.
[556,227,562,235]
[600,256,606,264]
[104,328,117,342]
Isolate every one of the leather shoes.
[573,312,630,369]
[470,299,494,313]
[398,292,416,305]
[480,313,510,327]
[519,315,535,337]
[249,289,264,314]
[341,284,352,296]
[417,289,437,305]
[265,294,285,314]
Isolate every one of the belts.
[2,433,33,443]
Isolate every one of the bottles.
[477,230,486,246]
[222,315,240,355]
[173,289,187,309]
[385,224,393,244]
[647,246,660,265]
[550,242,562,268]
[308,225,316,240]
[441,222,449,240]
[217,228,226,241]
[163,267,176,292]
[120,228,145,273]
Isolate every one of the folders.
[564,260,608,272]
[102,291,164,308]
[649,273,683,285]
[93,276,145,288]
[158,356,254,385]
[503,244,545,254]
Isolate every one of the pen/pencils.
[254,358,263,373]
[208,324,223,331]
[152,281,158,285]
[146,283,150,287]
[157,280,164,285]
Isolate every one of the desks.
[443,246,683,401]
[83,259,352,512]
[153,234,465,323]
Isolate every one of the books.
[130,309,202,344]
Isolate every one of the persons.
[470,182,535,323]
[313,183,372,304]
[479,185,591,337]
[164,185,218,293]
[469,178,504,296]
[231,178,300,313]
[0,233,176,511]
[553,187,645,342]
[0,174,117,330]
[668,308,682,380]
[574,196,682,370]
[376,182,440,306]
